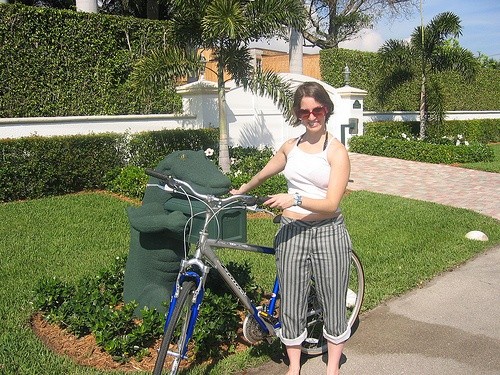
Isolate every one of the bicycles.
[145,169,365,375]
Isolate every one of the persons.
[228,82,352,375]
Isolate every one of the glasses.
[297,106,326,119]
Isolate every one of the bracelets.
[294,192,302,206]
